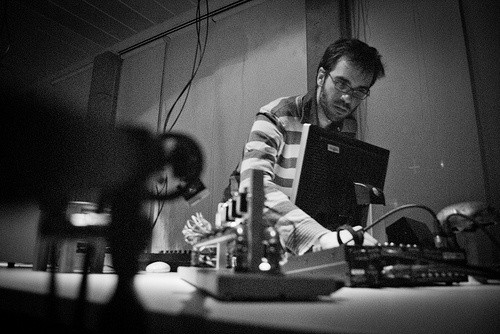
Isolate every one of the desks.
[0,266,500,334]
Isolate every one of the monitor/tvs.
[290,123,390,246]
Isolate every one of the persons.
[215,37,386,257]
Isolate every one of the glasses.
[328,74,370,100]
